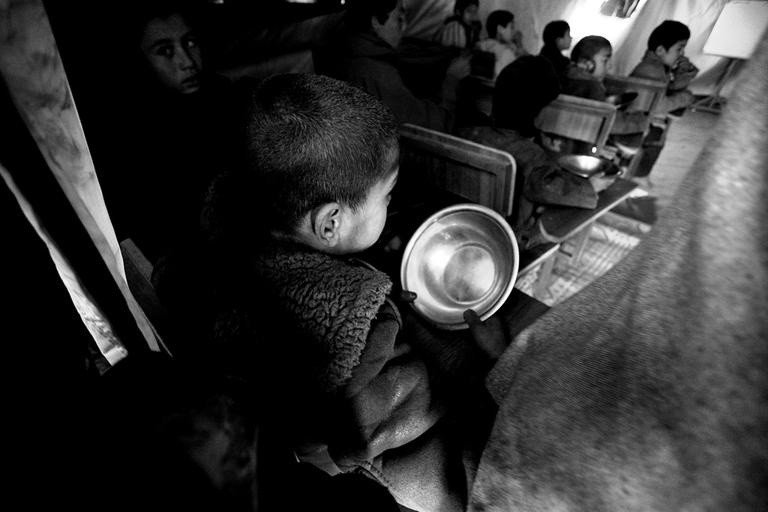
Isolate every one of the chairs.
[396,121,558,308]
[597,71,668,192]
[540,94,620,268]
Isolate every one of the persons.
[153,72,516,509]
[122,1,696,194]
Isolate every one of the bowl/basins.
[555,153,623,197]
[399,202,521,333]
